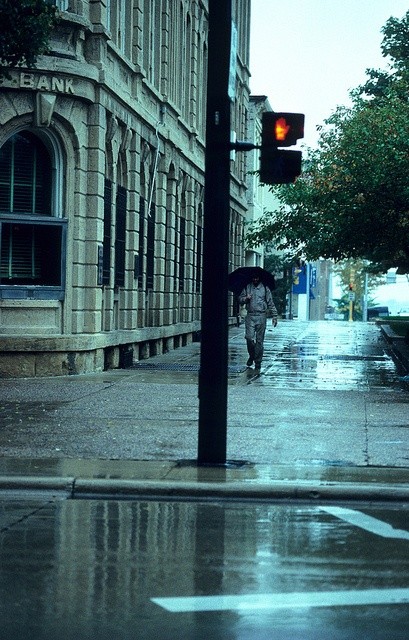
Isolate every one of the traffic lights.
[260,112,304,183]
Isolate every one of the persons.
[239,273,279,370]
[237,324,239,324]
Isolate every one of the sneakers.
[247,357,253,366]
[255,362,261,370]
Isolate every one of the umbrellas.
[228,266,276,298]
